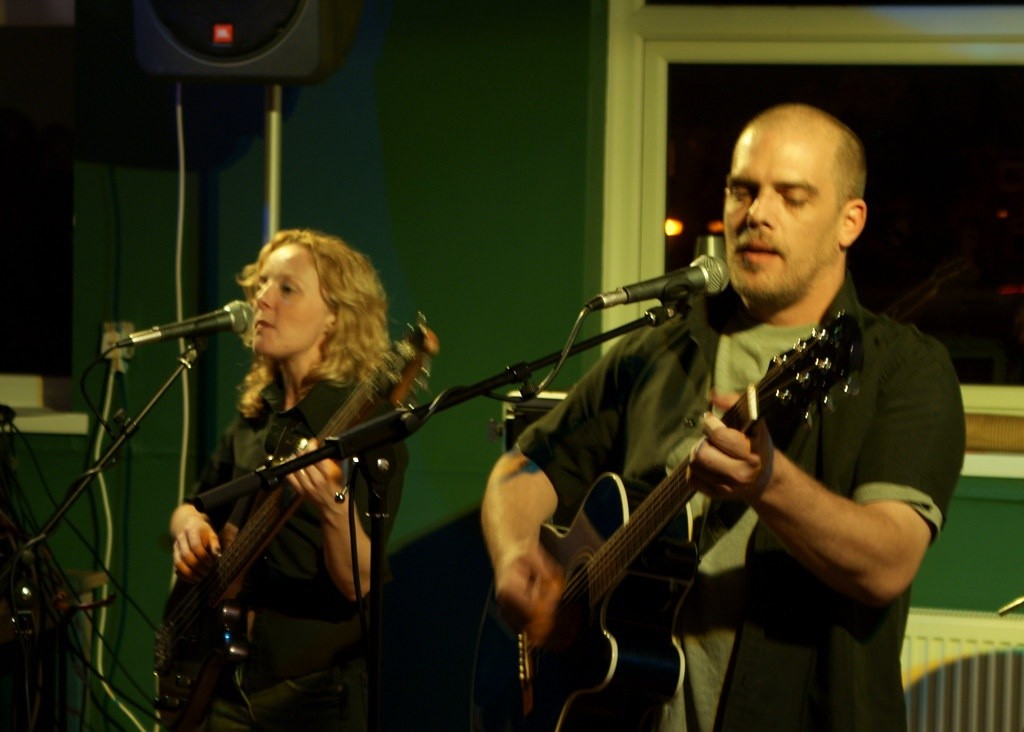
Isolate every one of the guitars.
[469,306,866,732]
[152,310,440,732]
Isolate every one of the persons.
[480,104,969,732]
[168,231,408,732]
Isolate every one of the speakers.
[133,0,324,84]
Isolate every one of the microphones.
[584,255,729,311]
[116,301,254,347]
